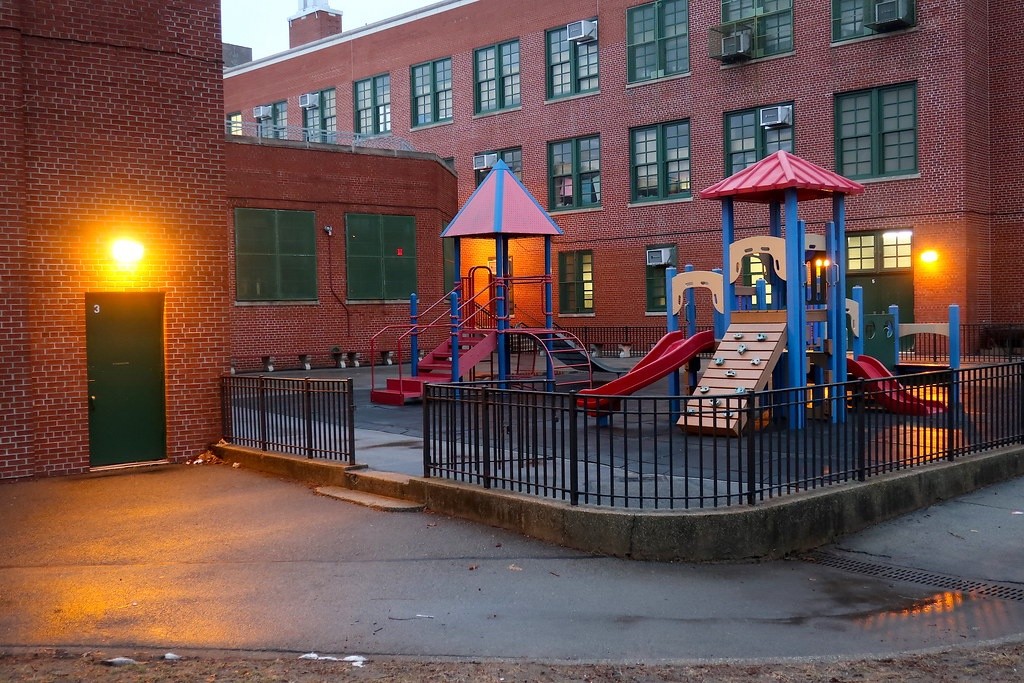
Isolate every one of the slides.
[531,333,632,374]
[846,354,948,416]
[577,330,714,417]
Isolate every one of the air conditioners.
[566,20,597,46]
[874,0,915,29]
[720,34,751,59]
[253,105,273,121]
[472,153,498,171]
[646,247,675,266]
[759,105,793,130]
[298,93,319,110]
